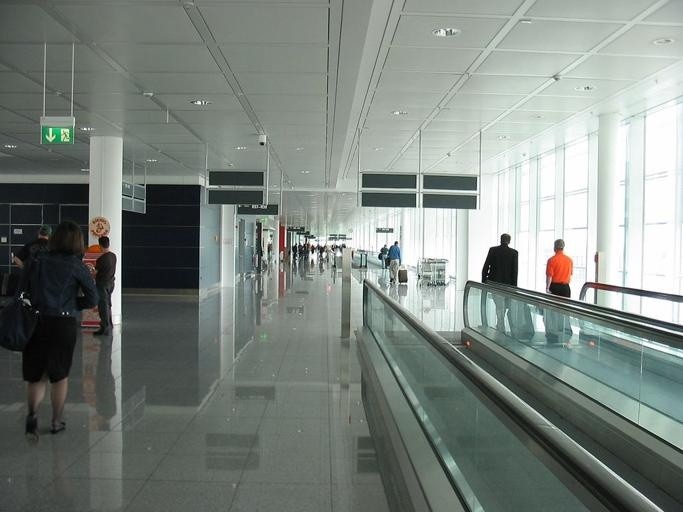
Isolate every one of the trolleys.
[419,259,451,287]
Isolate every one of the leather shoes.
[27,415,41,443]
[51,422,67,433]
[92,326,113,336]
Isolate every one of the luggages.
[399,269,408,282]
[543,309,573,345]
[507,299,535,340]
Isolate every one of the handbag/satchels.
[386,257,390,266]
[1,294,38,352]
[378,253,382,261]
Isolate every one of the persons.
[20,220,101,445]
[543,238,574,299]
[290,241,346,260]
[479,233,519,334]
[14,223,52,269]
[94,236,116,334]
[95,335,117,432]
[293,260,324,274]
[388,240,402,284]
[381,243,389,270]
